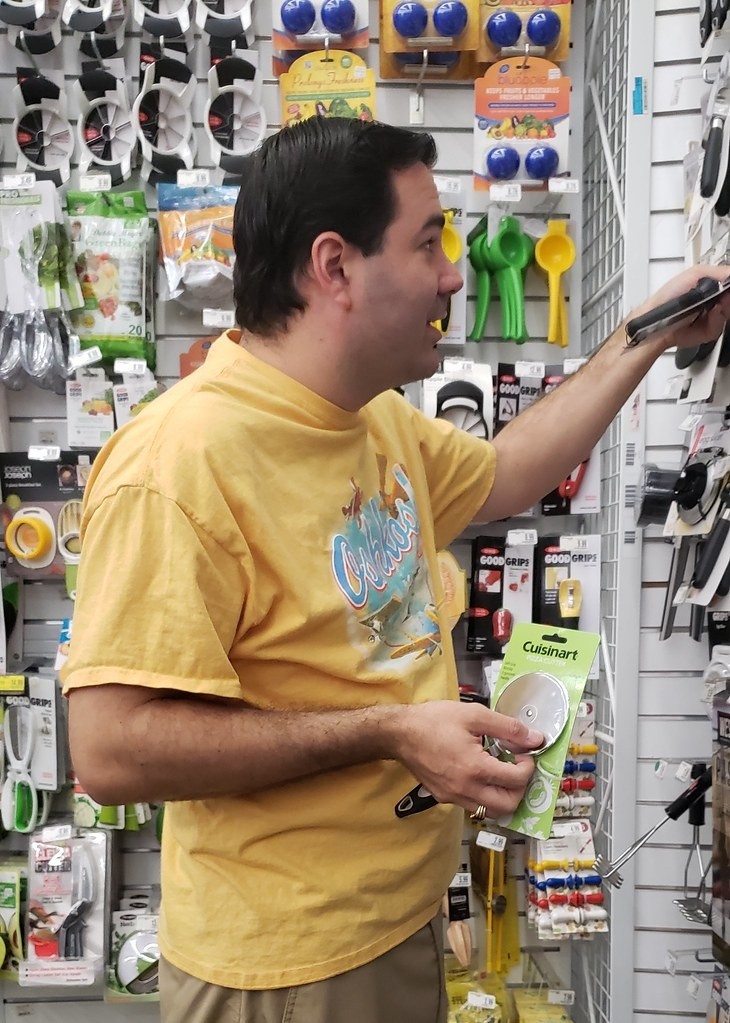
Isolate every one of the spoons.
[468,231,491,344]
[490,215,533,344]
[534,219,576,344]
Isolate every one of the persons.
[58,117,730,1023]
